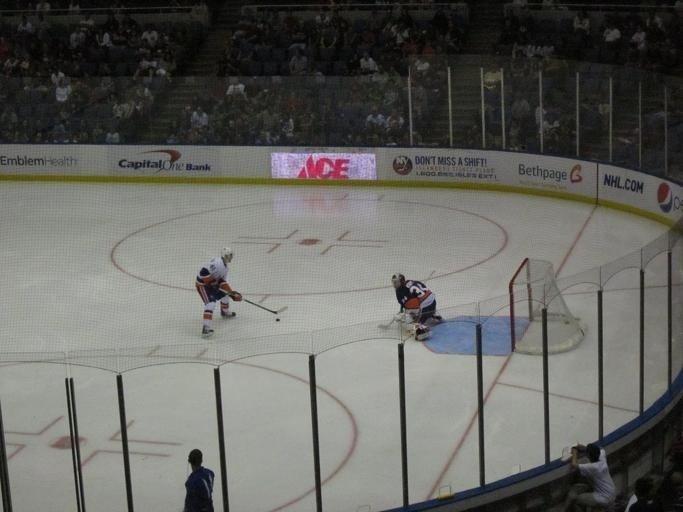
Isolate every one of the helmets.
[220,247,233,258]
[391,273,405,287]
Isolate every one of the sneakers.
[431,312,442,320]
[221,310,236,317]
[201,326,214,335]
[415,327,431,340]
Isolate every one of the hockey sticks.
[218,286,287,314]
[376,271,437,330]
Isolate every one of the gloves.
[229,290,243,302]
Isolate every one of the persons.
[1,0,683,186]
[193,246,242,335]
[183,448,214,512]
[631,450,682,512]
[391,272,441,338]
[563,442,617,512]
